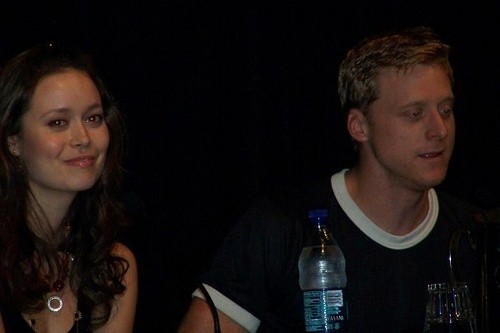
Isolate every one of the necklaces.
[39,255,75,312]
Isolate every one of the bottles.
[298,208,347,333]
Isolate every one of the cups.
[426,283,473,333]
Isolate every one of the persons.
[1,35,139,332]
[175,27,500,333]
